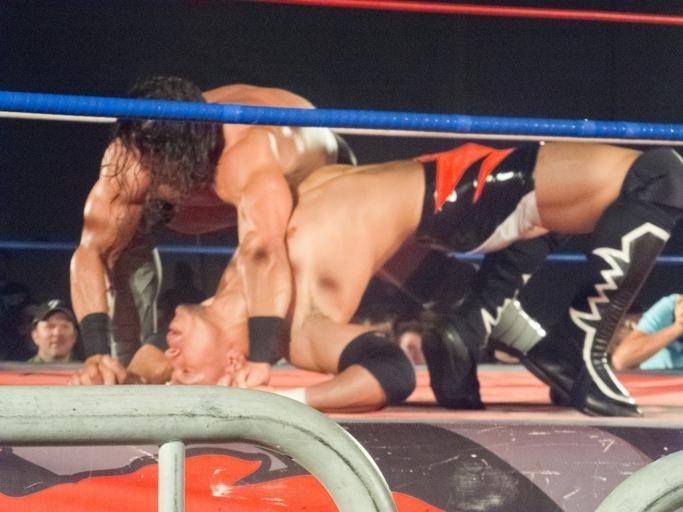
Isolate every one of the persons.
[392,318,429,368]
[62,137,682,418]
[64,73,576,407]
[611,286,682,372]
[20,296,84,367]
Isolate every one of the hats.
[28,296,74,326]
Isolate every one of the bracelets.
[77,311,112,361]
[243,314,289,367]
[265,385,311,407]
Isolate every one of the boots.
[524,205,677,418]
[423,232,553,413]
